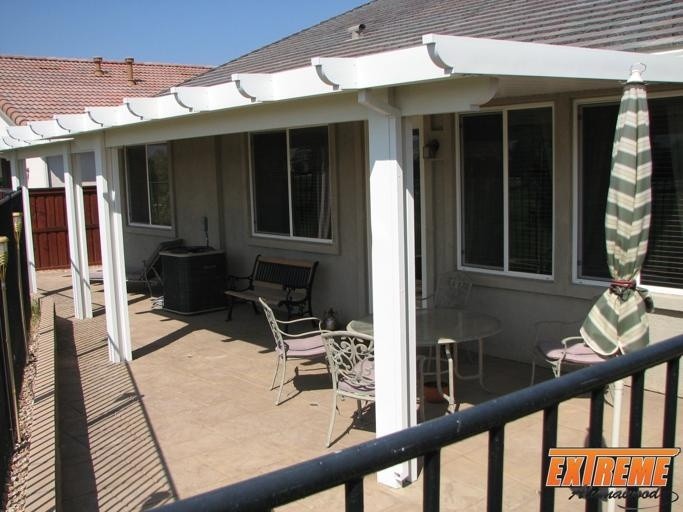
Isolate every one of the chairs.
[257,267,616,451]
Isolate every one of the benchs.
[220,254,322,337]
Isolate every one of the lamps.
[422,137,439,160]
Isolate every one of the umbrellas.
[576,62,655,511]
